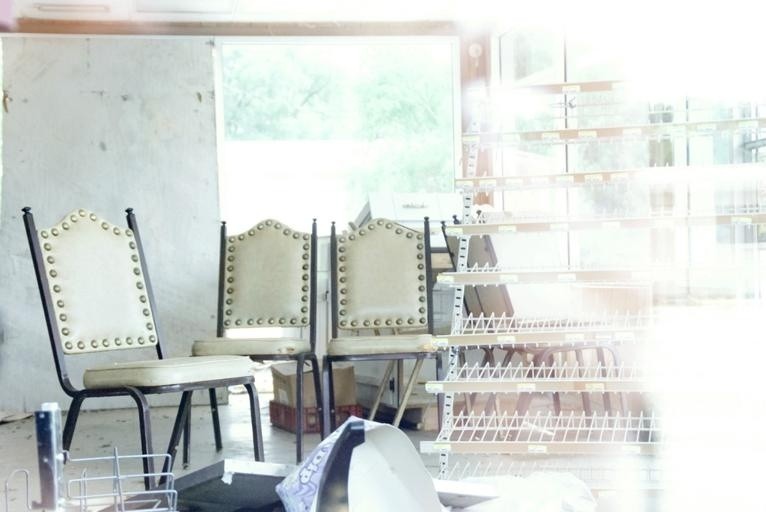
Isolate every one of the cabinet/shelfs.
[422,73,766,512]
